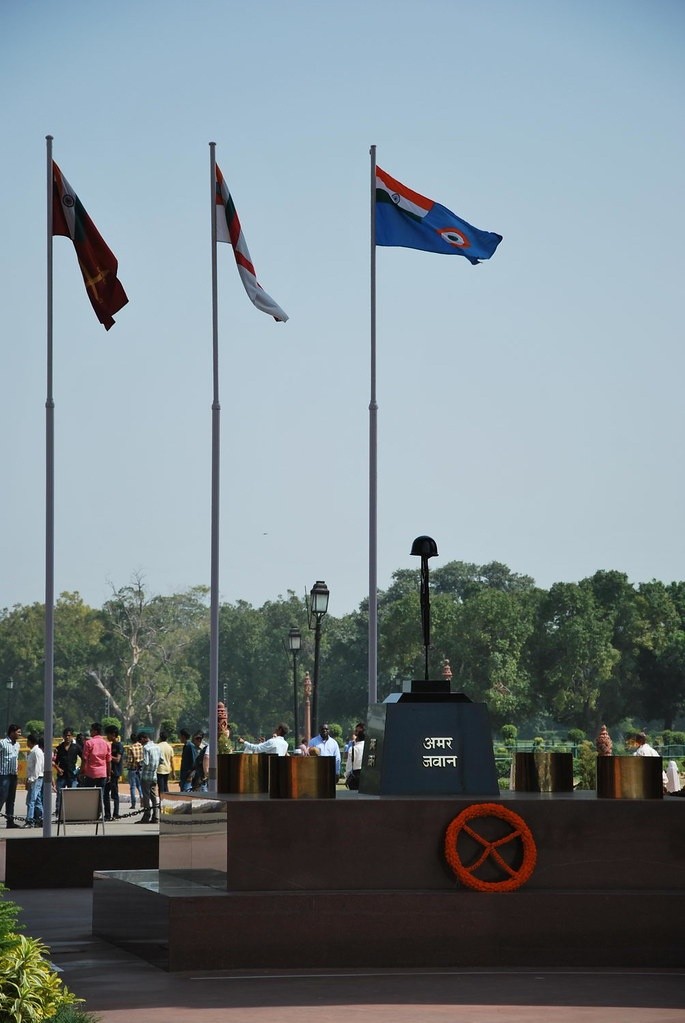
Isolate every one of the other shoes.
[6,819,20,828]
[23,823,32,828]
[149,819,158,823]
[99,816,111,820]
[134,820,150,824]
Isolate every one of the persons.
[237,721,368,791]
[629,732,668,790]
[0,722,207,828]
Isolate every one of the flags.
[375,165,503,266]
[52,160,130,331]
[215,164,288,323]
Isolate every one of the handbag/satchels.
[344,770,359,789]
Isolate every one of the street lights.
[309,580,330,738]
[6,676,14,729]
[287,625,302,756]
